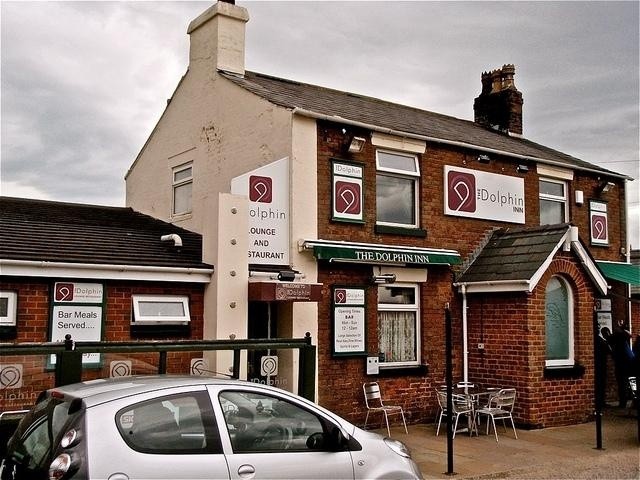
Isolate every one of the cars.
[0,374,425,478]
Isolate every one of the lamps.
[502,163,530,175]
[340,125,367,157]
[569,223,580,243]
[596,179,617,198]
[463,152,492,168]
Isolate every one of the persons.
[600,319,636,408]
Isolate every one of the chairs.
[432,381,520,445]
[362,381,410,437]
